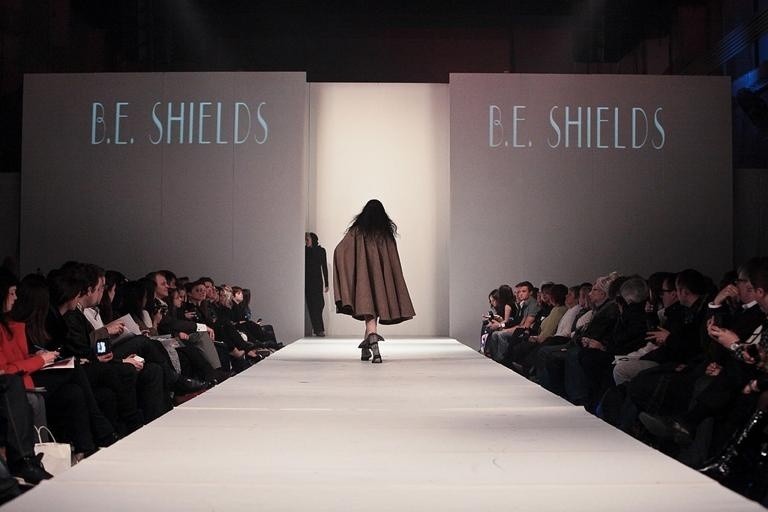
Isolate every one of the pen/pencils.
[34,345,65,360]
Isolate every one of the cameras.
[96,339,111,354]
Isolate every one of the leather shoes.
[639,412,690,449]
[173,374,208,396]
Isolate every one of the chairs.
[479,303,768,511]
[1,318,281,504]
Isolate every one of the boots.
[358,332,385,363]
[698,407,767,483]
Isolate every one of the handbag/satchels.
[33,424,72,476]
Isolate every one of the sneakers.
[9,453,54,486]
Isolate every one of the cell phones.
[746,344,760,362]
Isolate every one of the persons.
[0,261,284,503]
[304,233,329,337]
[480,263,768,506]
[333,199,417,363]
